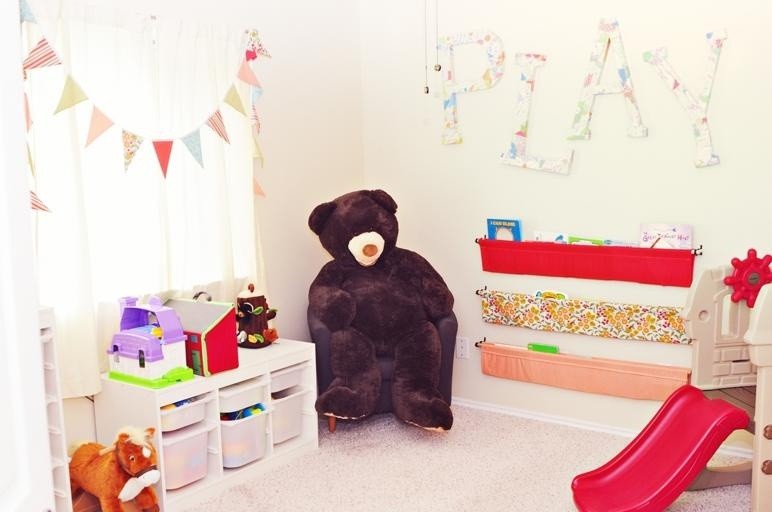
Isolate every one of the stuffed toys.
[306,189,458,432]
[69,427,161,512]
[235,284,279,349]
